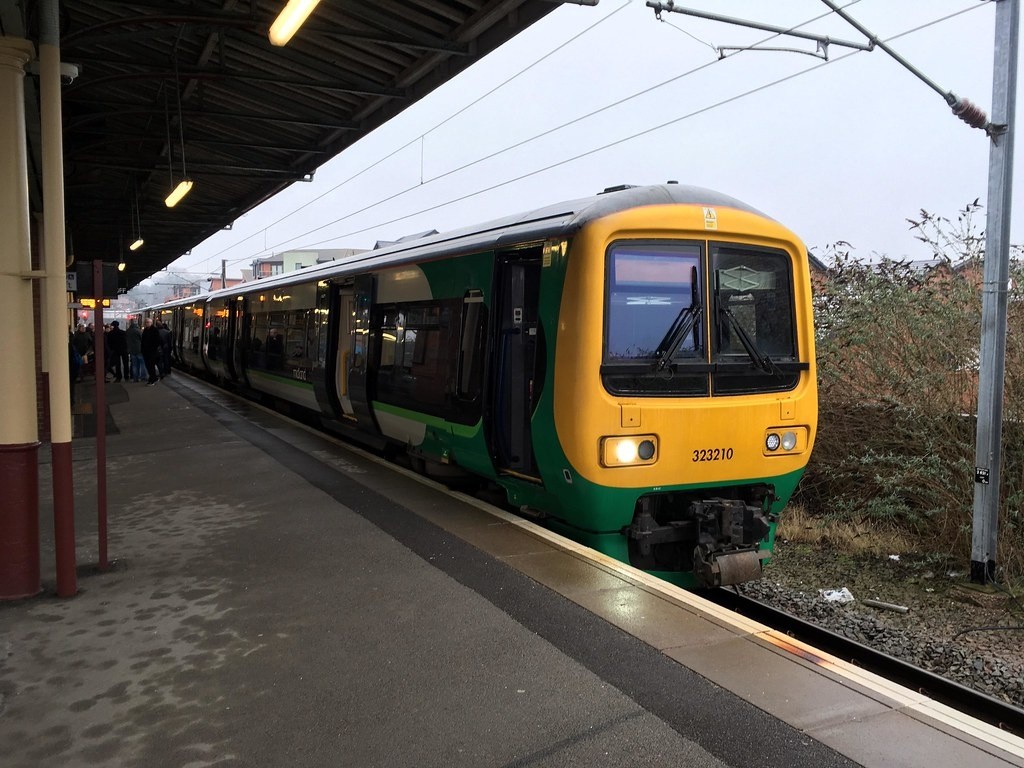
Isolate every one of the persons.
[189,320,293,399]
[68,315,175,391]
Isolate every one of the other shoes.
[106,372,174,386]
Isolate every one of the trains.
[127,181,819,589]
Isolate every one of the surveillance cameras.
[60,62,78,86]
